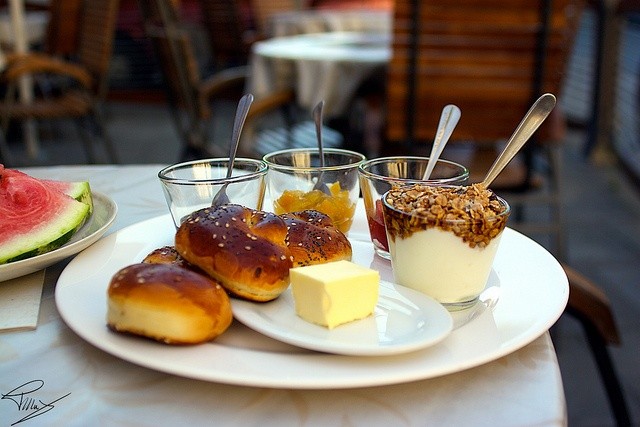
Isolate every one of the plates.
[0,191,118,282]
[54,201,570,388]
[231,281,454,355]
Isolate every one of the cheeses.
[290,258,378,329]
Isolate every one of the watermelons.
[0,164,95,262]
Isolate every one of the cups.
[263,148,366,235]
[358,157,467,264]
[158,159,269,233]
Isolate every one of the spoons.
[313,100,331,197]
[213,94,254,208]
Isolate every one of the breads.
[165,203,352,302]
[107,245,234,345]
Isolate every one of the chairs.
[364,0,588,265]
[146,0,345,170]
[0,0,122,166]
